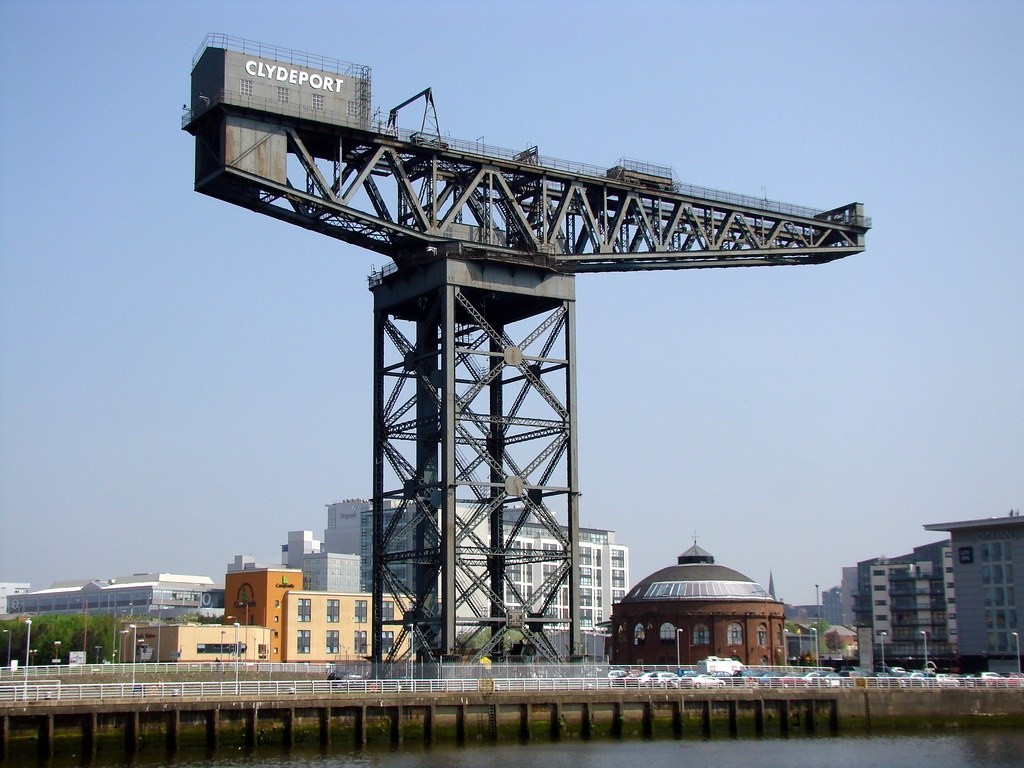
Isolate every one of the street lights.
[233,621,241,695]
[75,599,88,651]
[880,632,887,673]
[119,630,129,677]
[238,601,248,661]
[811,627,820,690]
[29,650,37,666]
[221,630,226,662]
[1010,631,1020,675]
[23,619,32,700]
[2,629,12,670]
[920,630,928,668]
[784,628,789,674]
[227,615,237,657]
[54,640,61,659]
[130,624,137,698]
[675,627,683,677]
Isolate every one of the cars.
[327,672,363,687]
[604,655,1024,689]
